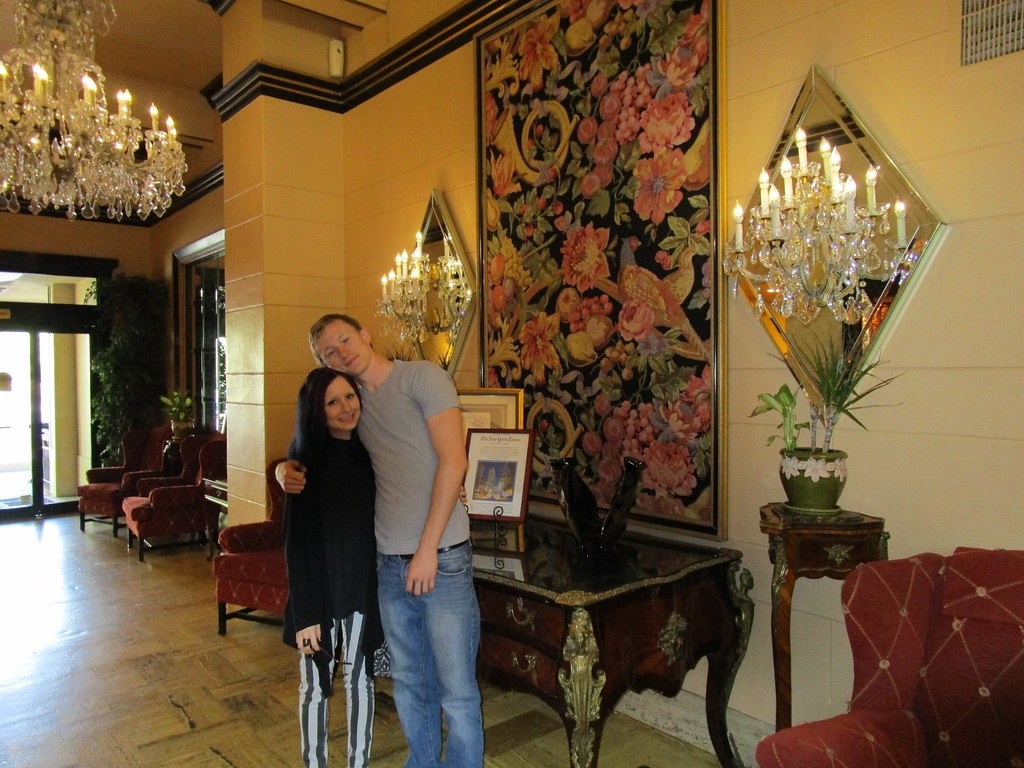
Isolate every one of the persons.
[282,367,384,768]
[276,314,484,768]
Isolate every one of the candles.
[444,236,450,256]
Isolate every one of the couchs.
[123,433,226,561]
[76,423,174,537]
[212,457,287,634]
[756,544,1024,767]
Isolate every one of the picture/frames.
[456,385,524,447]
[462,426,536,522]
[473,0,731,543]
[468,521,527,584]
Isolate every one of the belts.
[401,539,469,559]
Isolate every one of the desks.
[467,512,755,767]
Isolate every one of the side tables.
[759,503,892,733]
[202,474,227,561]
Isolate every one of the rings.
[303,638,310,647]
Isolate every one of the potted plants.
[160,391,197,439]
[748,325,904,515]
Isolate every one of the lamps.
[721,63,948,431]
[374,188,477,379]
[0,0,188,222]
